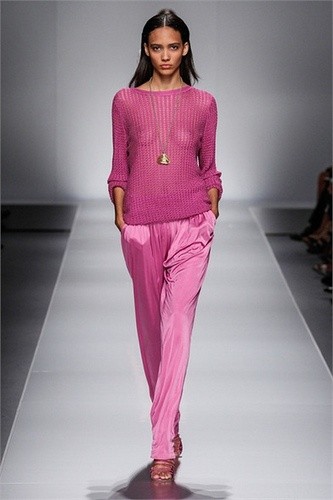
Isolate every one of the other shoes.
[288,225,333,293]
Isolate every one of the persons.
[289,163,333,293]
[107,9,223,482]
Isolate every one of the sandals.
[150,436,184,482]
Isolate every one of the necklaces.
[149,76,182,165]
